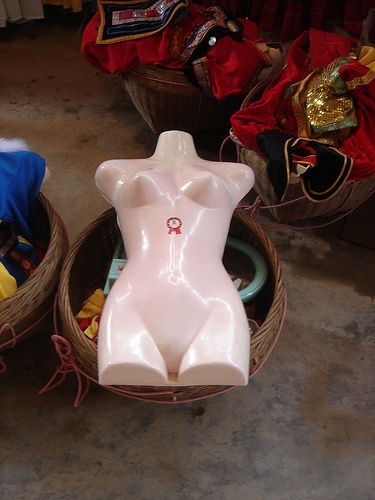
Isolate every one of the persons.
[95,131,254,386]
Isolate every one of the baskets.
[238,147,375,224]
[0,193,69,350]
[58,206,286,403]
[121,62,237,136]
